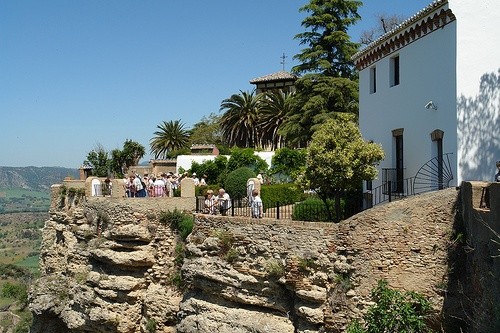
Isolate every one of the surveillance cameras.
[425,100,432,110]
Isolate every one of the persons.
[257,170,266,185]
[123,171,207,197]
[205,189,217,215]
[103,179,112,196]
[485,161,500,210]
[215,189,232,216]
[251,190,262,218]
